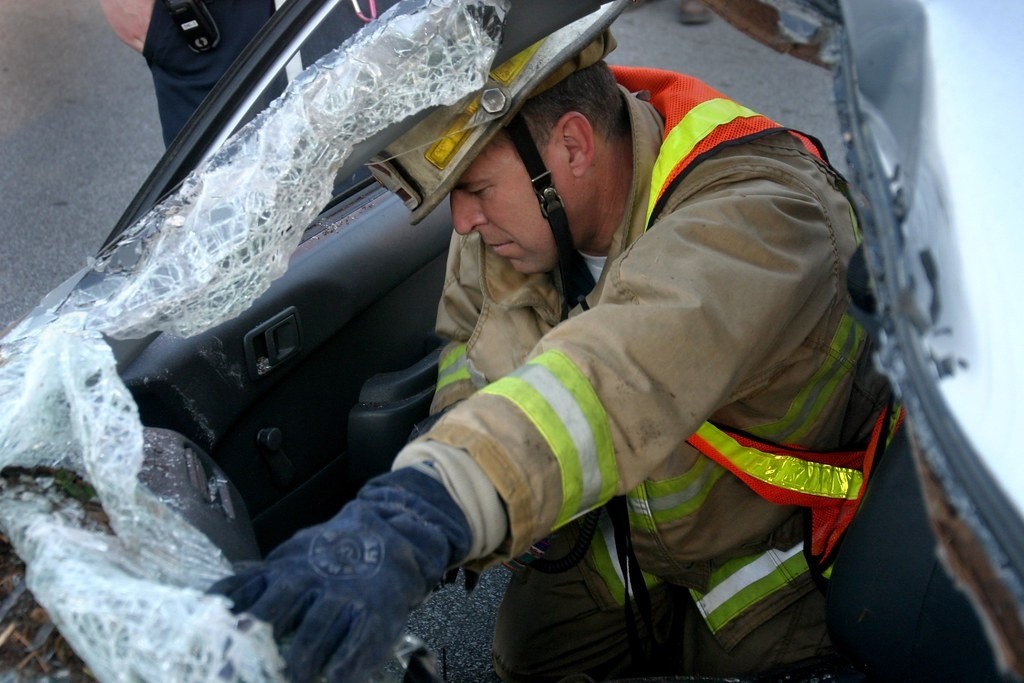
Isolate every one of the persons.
[100,0,284,150]
[200,27,906,682]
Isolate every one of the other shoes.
[679,0,712,24]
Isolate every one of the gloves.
[202,459,473,683]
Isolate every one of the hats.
[290,0,634,227]
